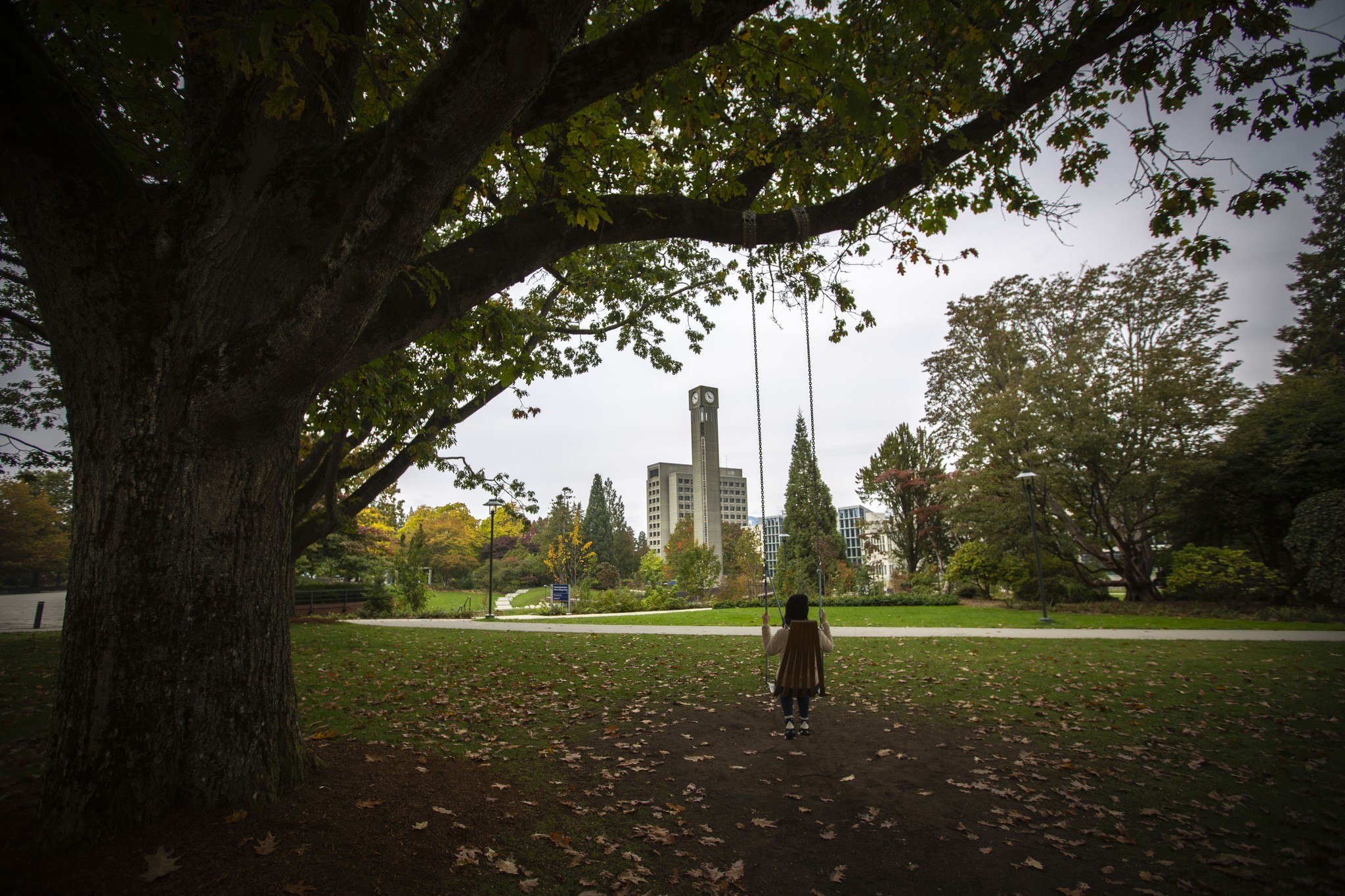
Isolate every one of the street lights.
[658,572,661,585]
[1014,466,1053,625]
[644,570,648,587]
[482,499,503,618]
[778,532,790,572]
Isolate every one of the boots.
[784,718,796,740]
[798,718,810,736]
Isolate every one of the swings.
[739,205,825,700]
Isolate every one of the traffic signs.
[552,584,568,601]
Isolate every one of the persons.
[761,594,834,740]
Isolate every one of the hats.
[786,594,809,619]
